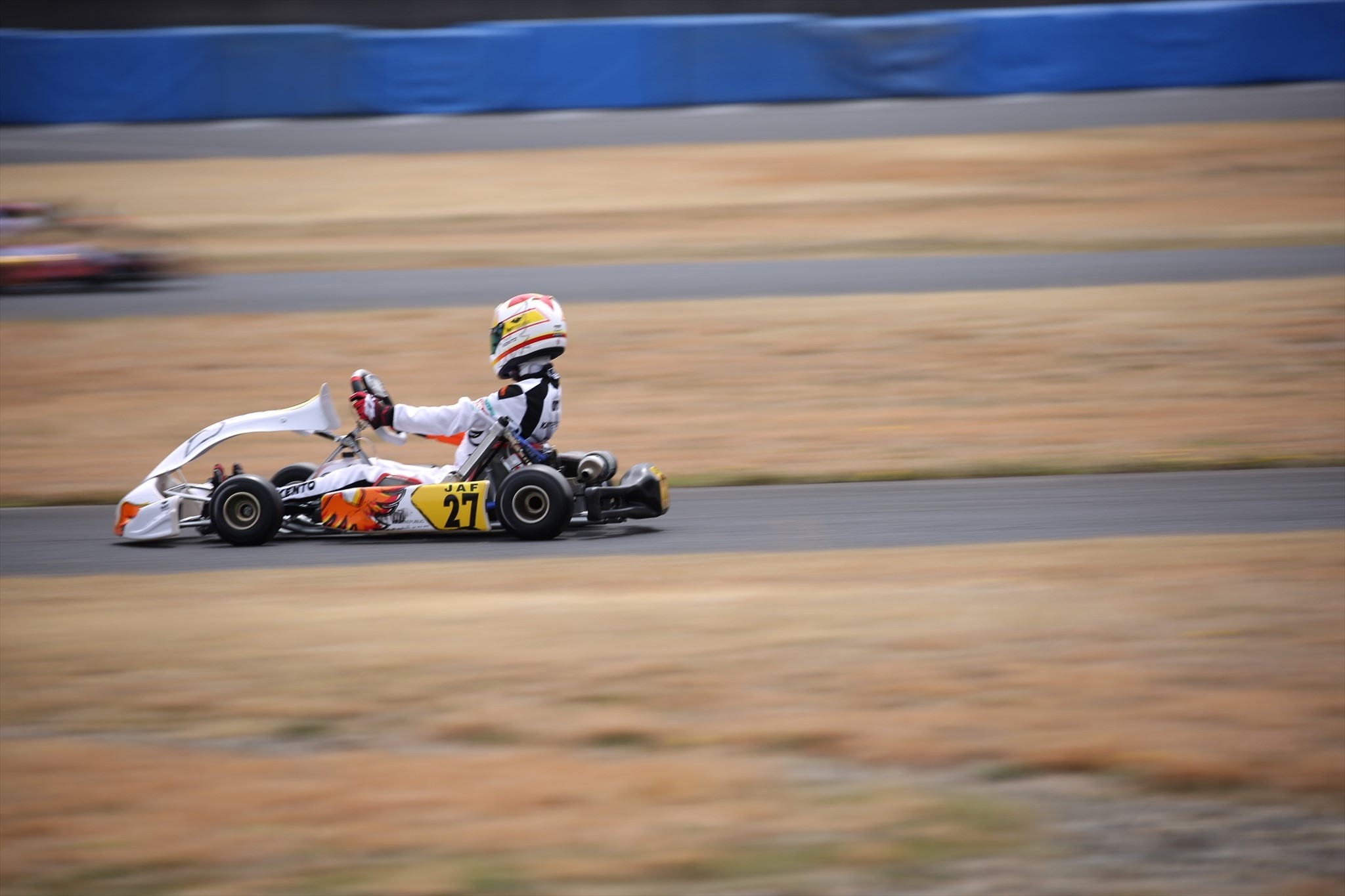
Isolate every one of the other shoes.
[234,463,243,475]
[212,462,225,483]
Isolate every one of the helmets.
[488,293,568,379]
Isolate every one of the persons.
[212,292,568,514]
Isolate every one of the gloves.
[349,392,394,430]
